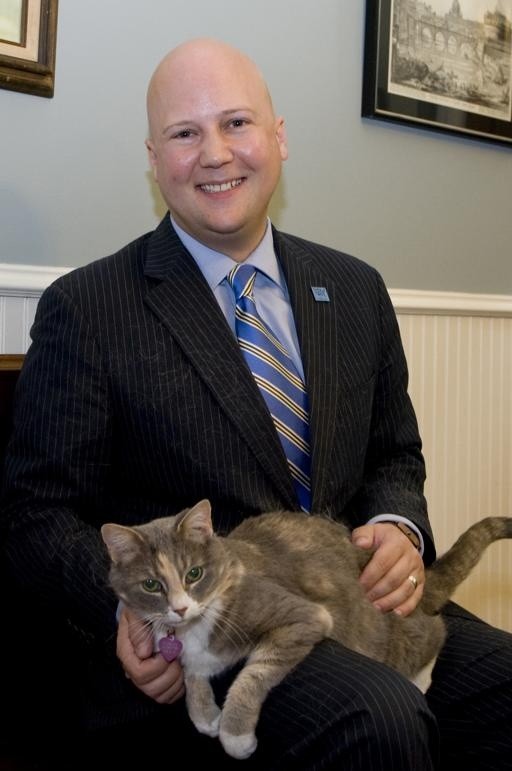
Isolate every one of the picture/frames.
[0,0,58,98]
[361,0,512,148]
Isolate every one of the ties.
[223,262,312,519]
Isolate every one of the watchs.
[376,521,420,551]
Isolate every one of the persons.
[0,35,512,771]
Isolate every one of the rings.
[125,671,131,679]
[409,575,417,588]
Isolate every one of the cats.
[101,498,511,759]
